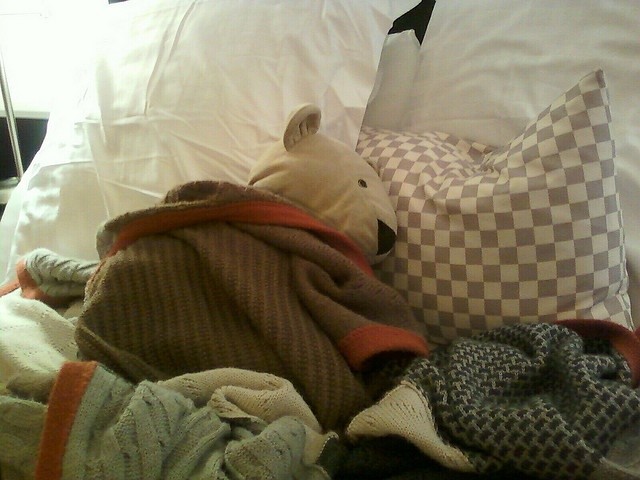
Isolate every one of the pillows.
[360,25,420,129]
[1,0,383,305]
[349,68,637,340]
[390,1,637,327]
